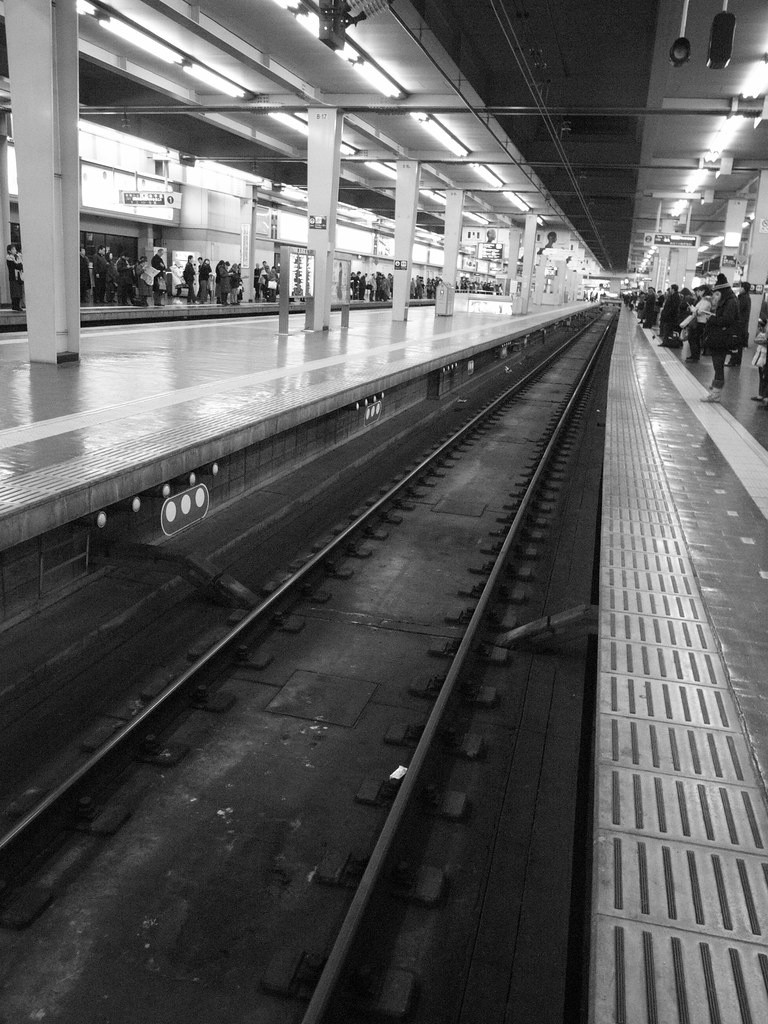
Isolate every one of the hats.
[713,273,733,300]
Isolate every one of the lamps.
[73,0,547,229]
[668,0,737,69]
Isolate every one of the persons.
[621,272,768,408]
[536,248,546,255]
[0,242,505,332]
[565,255,573,267]
[544,231,557,249]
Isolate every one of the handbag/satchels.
[268,281,277,290]
[687,316,697,332]
[752,344,767,367]
[160,277,167,290]
[366,284,372,290]
[668,333,683,349]
[680,314,696,329]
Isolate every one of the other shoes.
[725,362,741,368]
[93,300,164,307]
[751,396,768,409]
[699,387,722,402]
[187,299,240,305]
[686,356,699,364]
[12,307,23,311]
